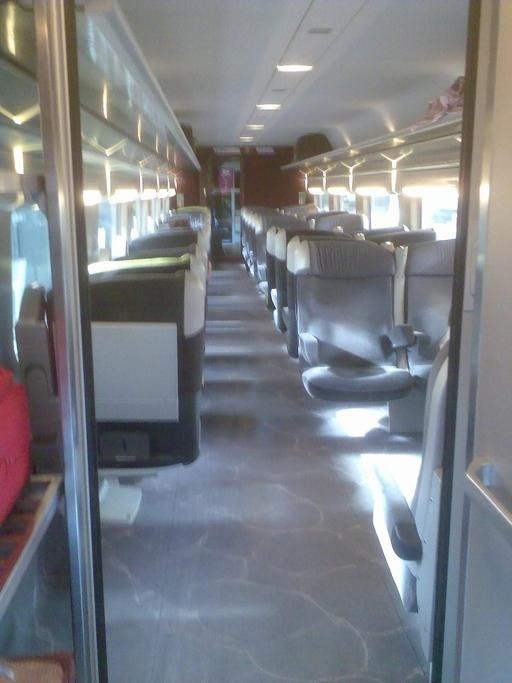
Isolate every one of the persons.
[170,218,212,284]
[305,203,319,215]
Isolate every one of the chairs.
[17,204,213,466]
[238,200,460,402]
